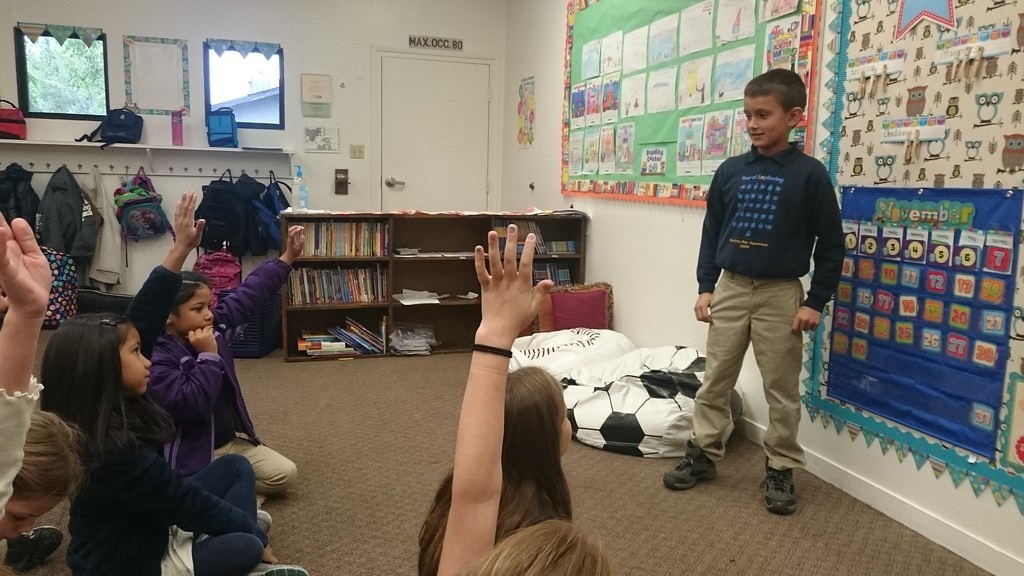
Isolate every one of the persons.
[39,192,311,575]
[662,68,847,516]
[151,223,305,535]
[1,208,82,576]
[432,224,613,576]
[419,365,576,576]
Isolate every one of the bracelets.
[473,344,514,360]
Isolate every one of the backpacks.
[191,250,242,314]
[36,246,80,330]
[193,169,248,262]
[249,170,293,250]
[113,166,176,246]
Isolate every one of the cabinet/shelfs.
[279,206,591,363]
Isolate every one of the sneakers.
[662,440,718,491]
[249,563,308,576]
[762,456,798,515]
[196,509,272,547]
[7,525,63,573]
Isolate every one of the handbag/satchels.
[218,287,282,360]
[89,107,144,144]
[204,107,239,148]
[1,99,27,140]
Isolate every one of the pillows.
[550,280,614,335]
[529,284,589,334]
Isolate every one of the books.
[287,221,389,258]
[296,314,387,357]
[392,260,574,306]
[288,264,388,307]
[388,320,443,356]
[392,219,578,255]
